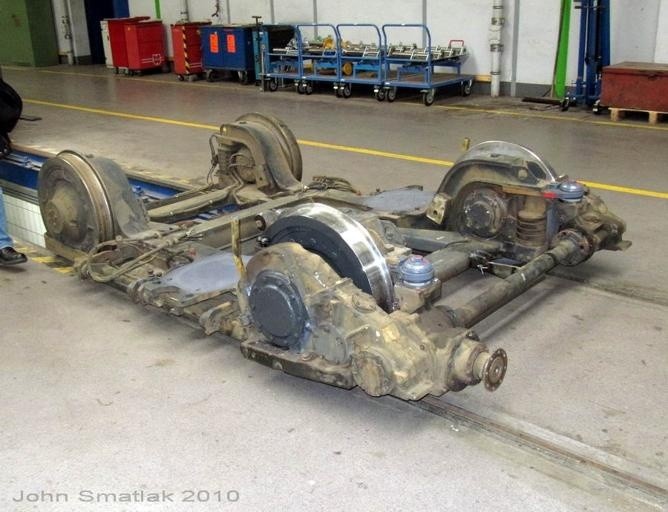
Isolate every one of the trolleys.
[107,15,470,106]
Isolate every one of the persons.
[0,67,28,268]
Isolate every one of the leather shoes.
[0,246,27,264]
